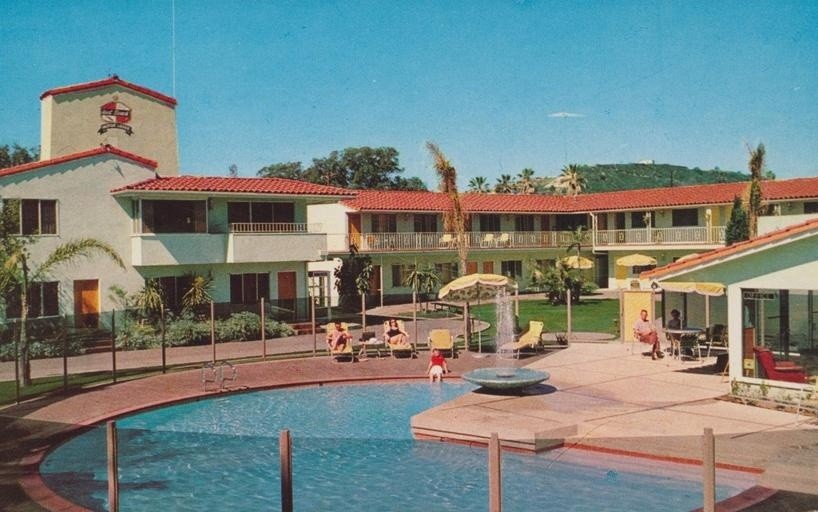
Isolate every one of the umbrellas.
[639,253,727,327]
[616,253,657,279]
[555,254,594,270]
[439,272,521,355]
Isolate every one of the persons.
[327,320,354,353]
[632,308,664,360]
[666,309,683,355]
[381,318,411,348]
[424,348,448,382]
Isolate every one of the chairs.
[500,320,544,360]
[326,319,454,363]
[632,322,728,364]
[752,349,808,383]
